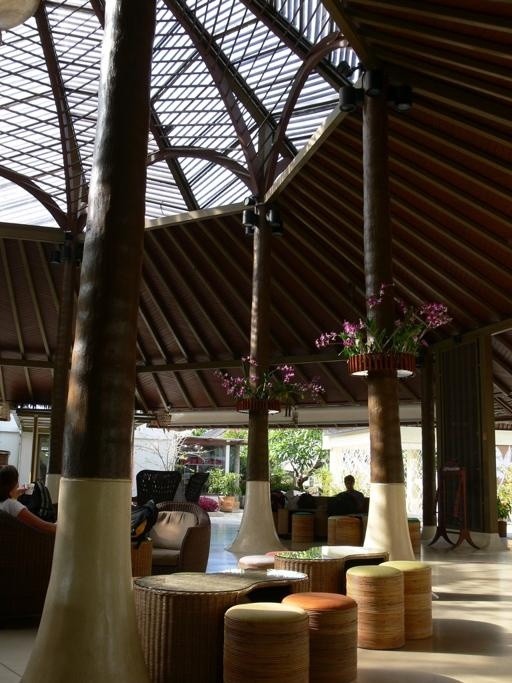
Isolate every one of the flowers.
[314,283,451,354]
[213,356,323,397]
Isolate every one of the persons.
[0,465,57,534]
[335,475,365,515]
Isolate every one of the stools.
[132,538,153,577]
[224,544,433,683]
[272,495,421,554]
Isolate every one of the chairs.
[184,473,209,504]
[136,469,182,506]
[140,502,210,572]
[0,511,55,627]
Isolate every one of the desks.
[132,568,309,683]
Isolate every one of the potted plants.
[496,495,511,538]
[217,473,242,512]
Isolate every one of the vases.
[347,354,415,379]
[235,395,281,413]
